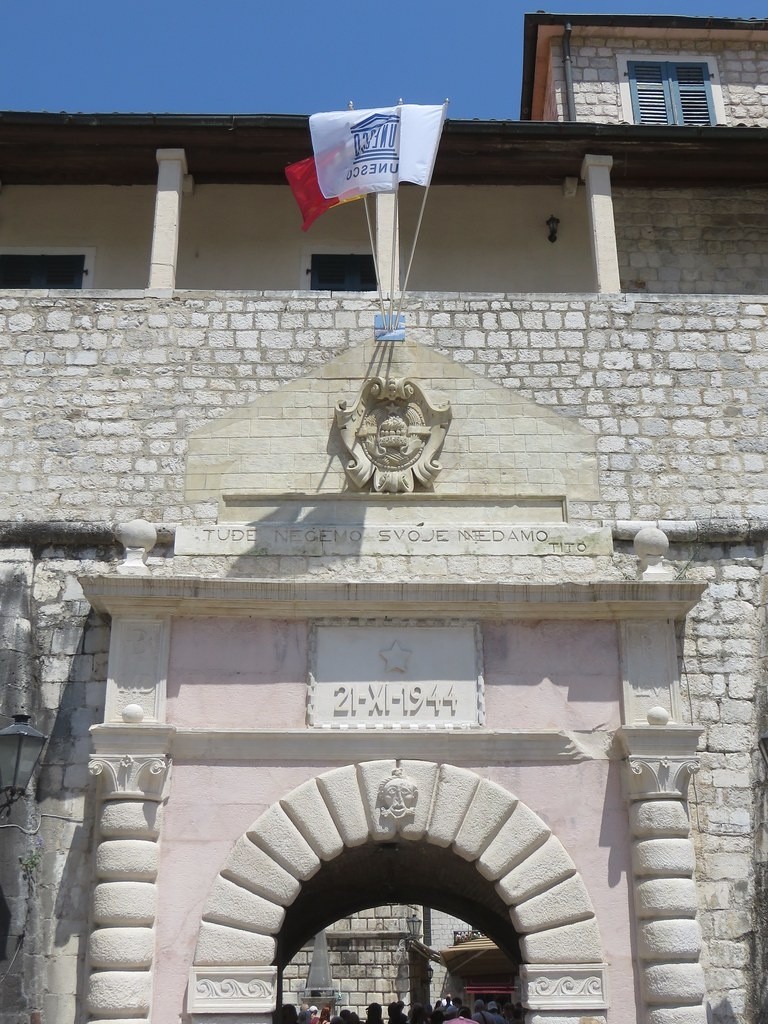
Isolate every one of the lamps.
[546,213,560,243]
[0,705,49,818]
[405,913,424,941]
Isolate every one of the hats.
[366,1003,382,1012]
[445,994,451,999]
[307,1006,318,1012]
[487,1001,498,1010]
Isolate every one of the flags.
[283,134,364,231]
[308,103,447,198]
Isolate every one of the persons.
[452,929,481,946]
[296,996,528,1024]
